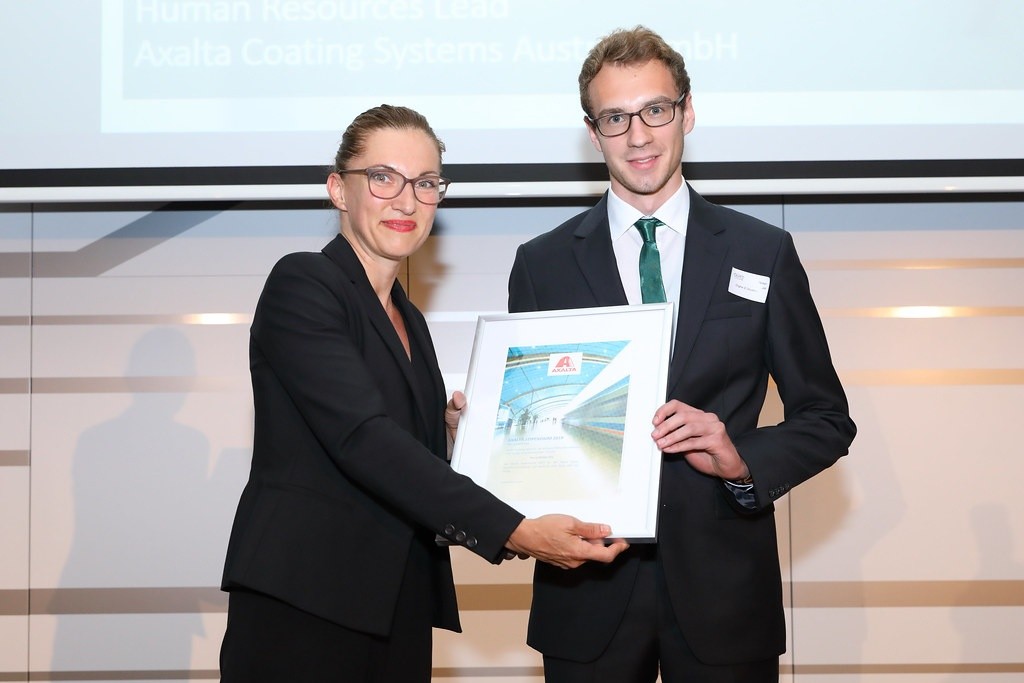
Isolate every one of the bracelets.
[728,481,754,492]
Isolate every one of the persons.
[220,105,627,683]
[508,29,858,682]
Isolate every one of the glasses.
[338,169,451,205]
[587,93,686,137]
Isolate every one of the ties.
[634,217,667,304]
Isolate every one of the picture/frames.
[437,303,675,543]
